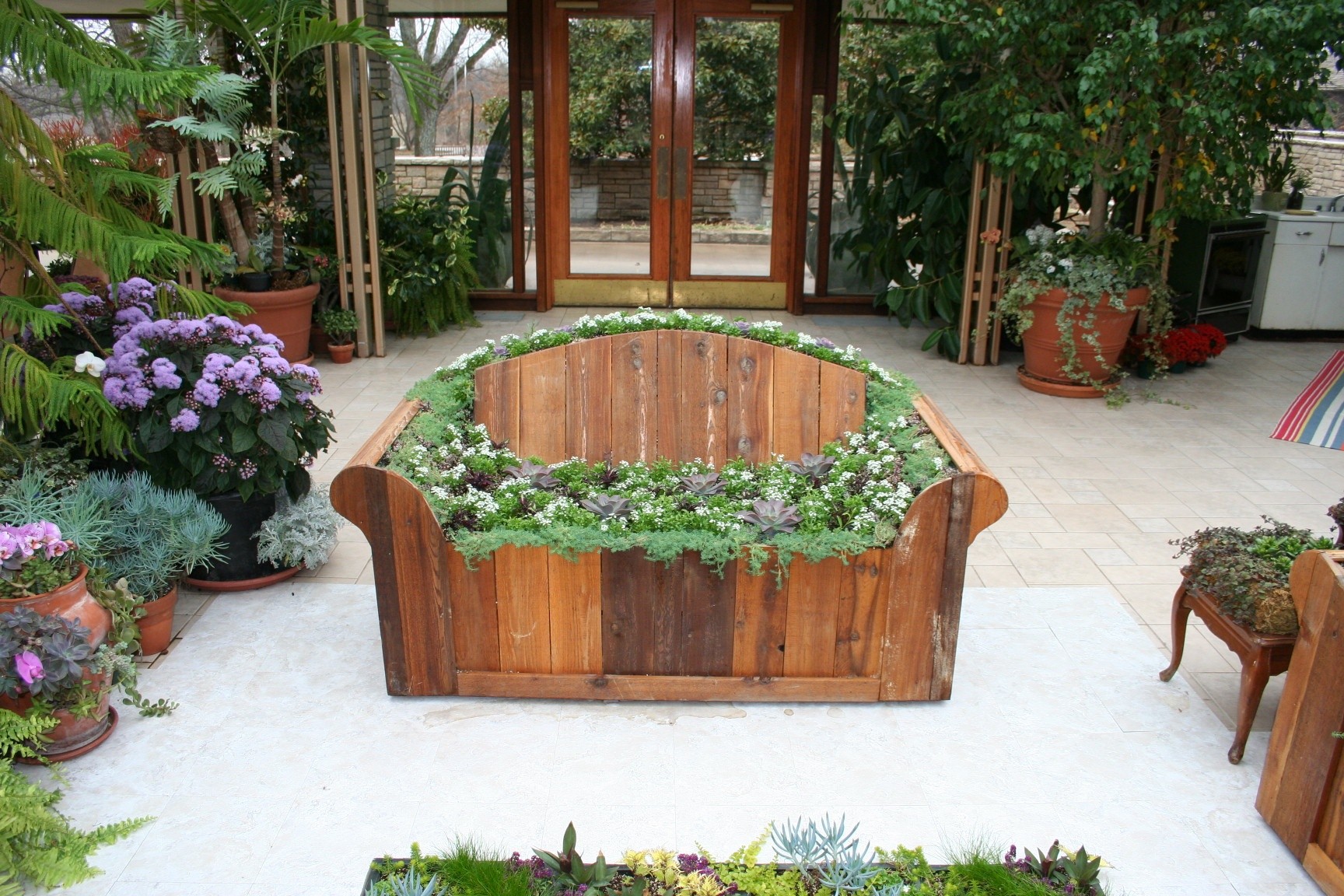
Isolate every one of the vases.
[329,327,1009,704]
[173,470,311,584]
[1138,346,1213,381]
[0,640,124,765]
[0,557,112,655]
[359,846,1102,896]
[1007,282,1147,396]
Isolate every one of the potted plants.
[189,1,450,366]
[316,308,360,364]
[1259,142,1300,209]
[1168,518,1328,638]
[1287,170,1312,210]
[68,471,231,657]
[436,101,514,290]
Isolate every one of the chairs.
[1160,567,1299,761]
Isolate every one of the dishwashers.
[1112,200,1272,338]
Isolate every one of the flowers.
[0,276,323,752]
[378,302,942,576]
[959,222,1183,413]
[1126,326,1226,367]
[365,810,1114,896]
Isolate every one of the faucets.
[1328,194,1344,212]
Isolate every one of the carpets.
[1268,347,1344,452]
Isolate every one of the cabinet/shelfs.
[1315,222,1344,329]
[1257,220,1333,329]
